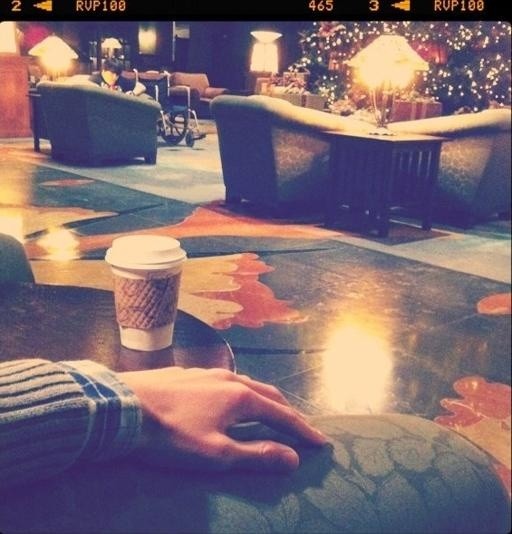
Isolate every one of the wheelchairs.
[133,68,207,147]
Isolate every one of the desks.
[0,281,237,371]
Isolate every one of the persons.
[87,57,147,98]
[1,355,330,480]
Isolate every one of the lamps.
[343,36,431,129]
[28,33,80,80]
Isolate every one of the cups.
[104,235,188,352]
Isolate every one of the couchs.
[25,71,512,228]
